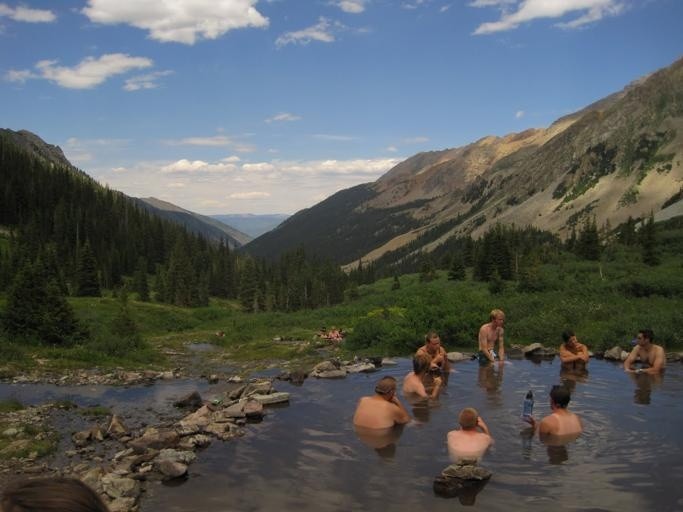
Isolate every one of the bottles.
[522,388,534,415]
[351,352,369,368]
[522,434,531,449]
[334,355,340,370]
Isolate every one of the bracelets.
[635,369,639,375]
[639,368,643,375]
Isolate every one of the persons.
[629,370,665,404]
[560,369,589,390]
[559,330,589,370]
[355,424,404,459]
[408,397,439,422]
[403,356,442,400]
[479,309,512,366]
[353,376,409,437]
[1,478,110,512]
[479,361,503,392]
[521,385,582,445]
[623,330,667,376]
[314,326,345,340]
[417,333,449,374]
[446,408,495,463]
[547,445,569,464]
